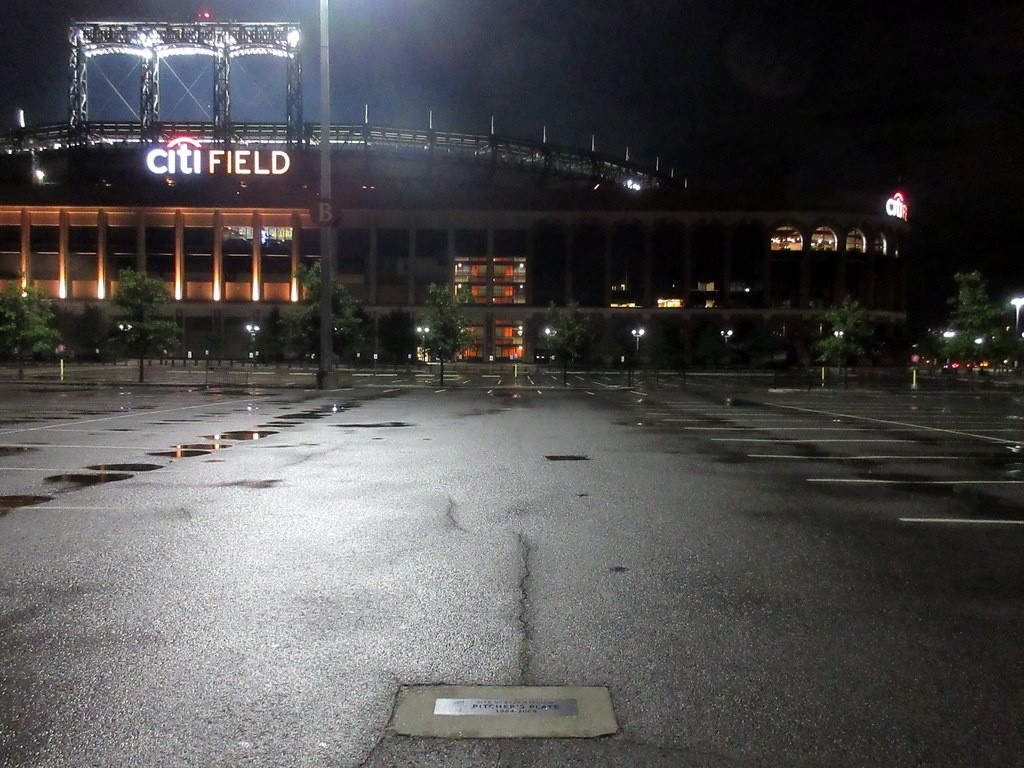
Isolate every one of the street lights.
[833,330,845,377]
[119,321,133,368]
[417,326,431,363]
[942,330,957,365]
[974,337,983,370]
[1011,296,1024,330]
[544,328,551,372]
[245,325,260,368]
[720,329,733,374]
[631,328,645,372]
